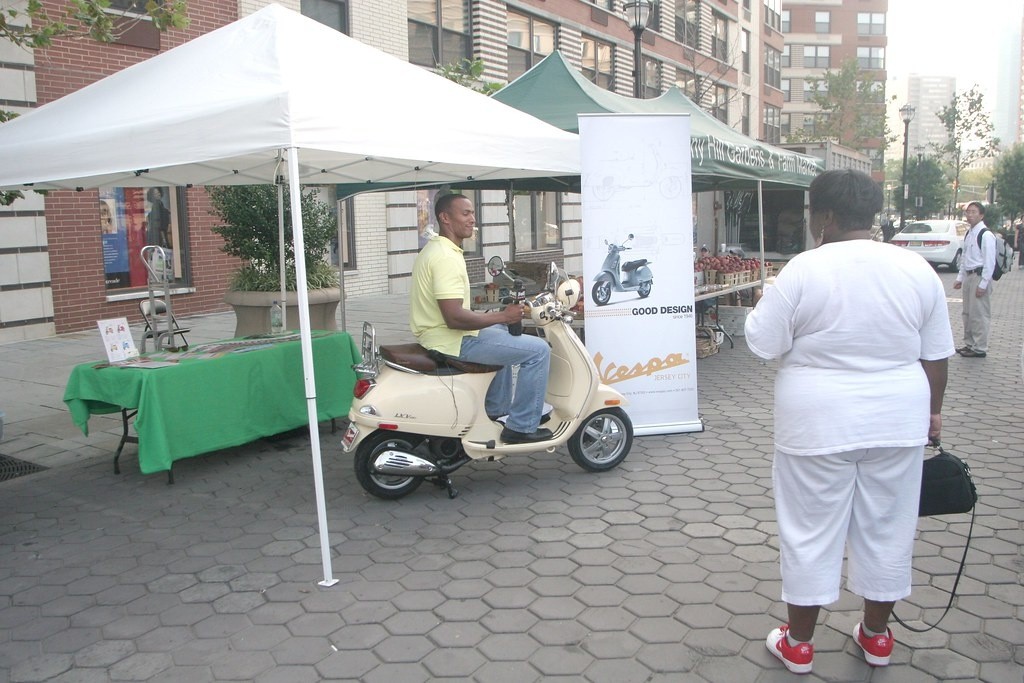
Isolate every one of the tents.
[483,47,827,365]
[0,0,580,588]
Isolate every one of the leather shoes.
[500,426,553,444]
[955,347,970,353]
[541,414,550,424]
[960,349,986,357]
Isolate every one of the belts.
[966,267,982,274]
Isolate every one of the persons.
[408,194,553,444]
[729,249,745,259]
[701,244,711,257]
[100,199,115,234]
[881,218,895,241]
[953,202,998,358]
[1013,214,1024,270]
[143,187,172,248]
[744,167,954,676]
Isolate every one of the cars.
[871,220,915,241]
[887,220,971,273]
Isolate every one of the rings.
[520,312,525,316]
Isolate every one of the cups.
[720,243,726,253]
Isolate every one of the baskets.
[696,327,719,358]
[703,265,773,292]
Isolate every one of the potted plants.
[204,184,351,338]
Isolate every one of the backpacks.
[964,227,1014,282]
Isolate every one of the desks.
[63,330,363,483]
[470,276,775,355]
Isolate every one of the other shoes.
[1019,265,1022,269]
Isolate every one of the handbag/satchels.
[891,441,978,520]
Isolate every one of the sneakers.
[852,617,894,667]
[766,623,814,674]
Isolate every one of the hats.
[700,245,711,253]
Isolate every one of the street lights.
[899,104,915,231]
[914,143,926,221]
[623,0,652,99]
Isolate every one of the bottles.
[508,300,523,337]
[270,300,283,335]
[701,243,708,258]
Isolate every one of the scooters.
[341,256,636,501]
[592,234,653,306]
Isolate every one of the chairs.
[139,298,191,348]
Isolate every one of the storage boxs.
[467,261,551,303]
[694,266,774,344]
[710,306,753,337]
[706,325,723,344]
